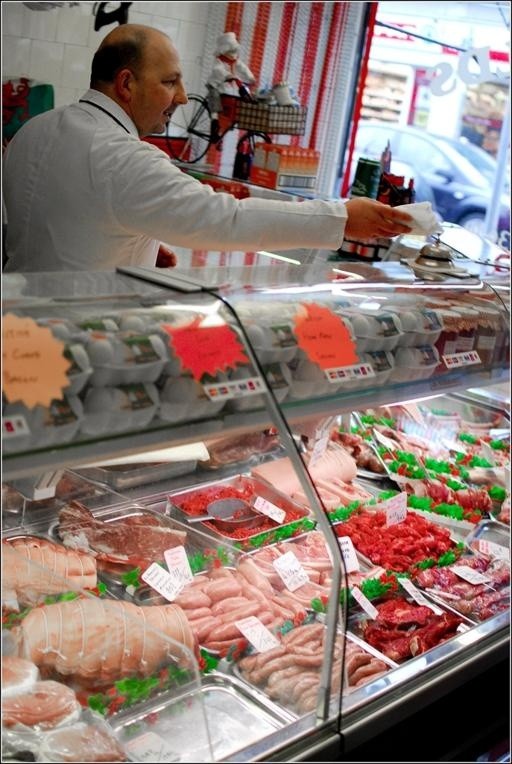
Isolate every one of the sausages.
[175,559,390,712]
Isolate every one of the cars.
[355,120,510,259]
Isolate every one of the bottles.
[233,139,253,181]
[336,157,418,263]
[235,79,253,102]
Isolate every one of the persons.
[207,31,257,143]
[3,23,415,273]
